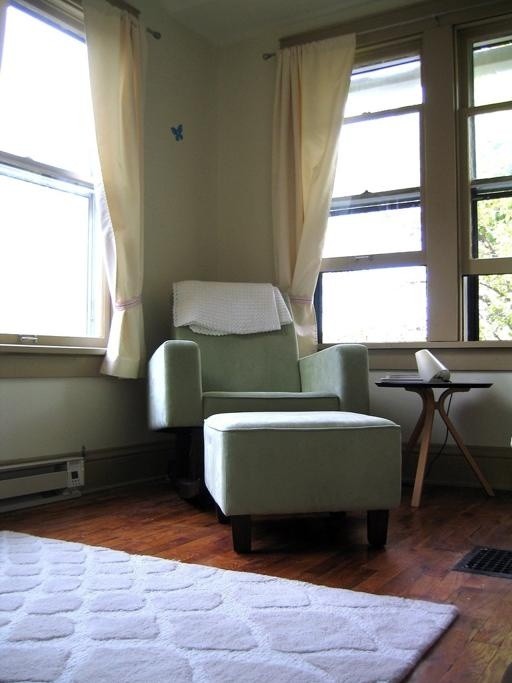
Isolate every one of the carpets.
[0,528,460,680]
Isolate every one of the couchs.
[200,406,404,555]
[147,275,371,499]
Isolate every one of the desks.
[372,377,494,510]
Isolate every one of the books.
[380,375,423,383]
